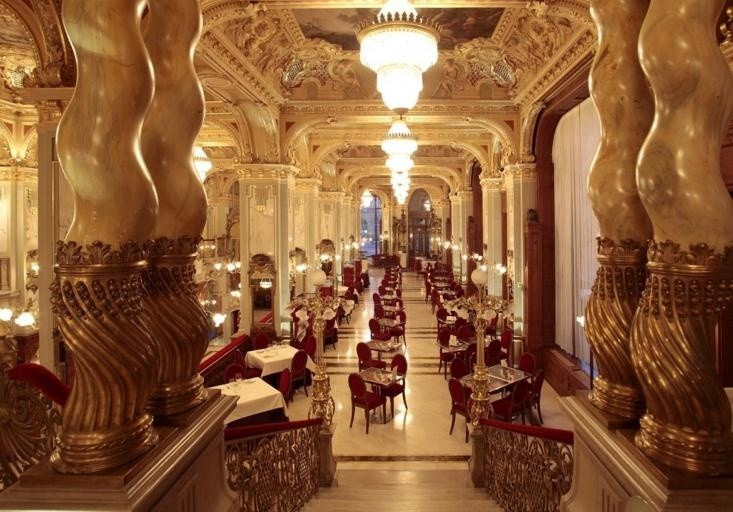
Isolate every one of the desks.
[435,336,489,361]
[459,364,531,422]
[358,369,403,423]
[366,340,401,368]
[244,345,322,378]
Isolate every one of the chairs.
[356,342,386,389]
[470,350,488,375]
[372,354,408,424]
[517,354,536,383]
[289,351,309,401]
[290,253,506,346]
[448,379,470,443]
[235,349,260,379]
[277,368,293,406]
[437,328,467,380]
[252,333,270,349]
[487,339,502,365]
[467,343,476,355]
[226,364,245,384]
[450,357,468,379]
[528,370,544,426]
[491,381,526,427]
[347,372,386,435]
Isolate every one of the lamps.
[259,270,272,290]
[351,0,443,205]
